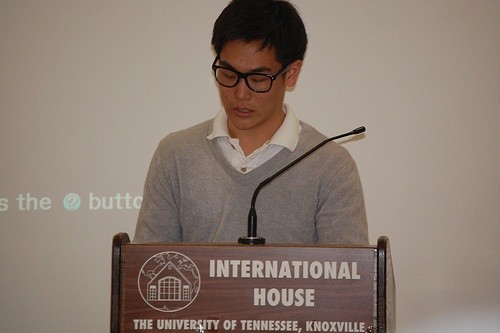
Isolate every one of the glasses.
[212,54,292,94]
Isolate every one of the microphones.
[238,125,366,245]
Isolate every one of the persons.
[130,0,370,249]
